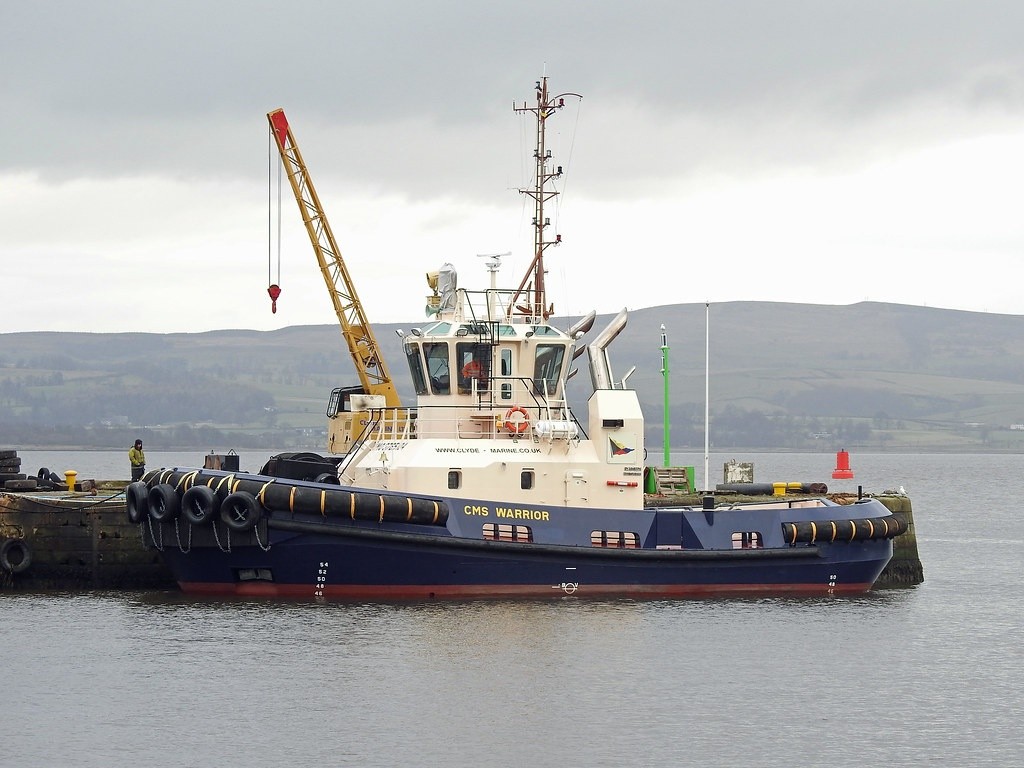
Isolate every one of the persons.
[128,439,145,483]
[461,353,484,390]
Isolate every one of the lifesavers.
[0,449,61,492]
[1,537,32,572]
[505,406,530,433]
[125,482,150,523]
[181,485,218,524]
[145,484,179,520]
[219,490,260,530]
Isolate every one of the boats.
[123,77,924,603]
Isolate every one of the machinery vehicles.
[263,107,419,455]
